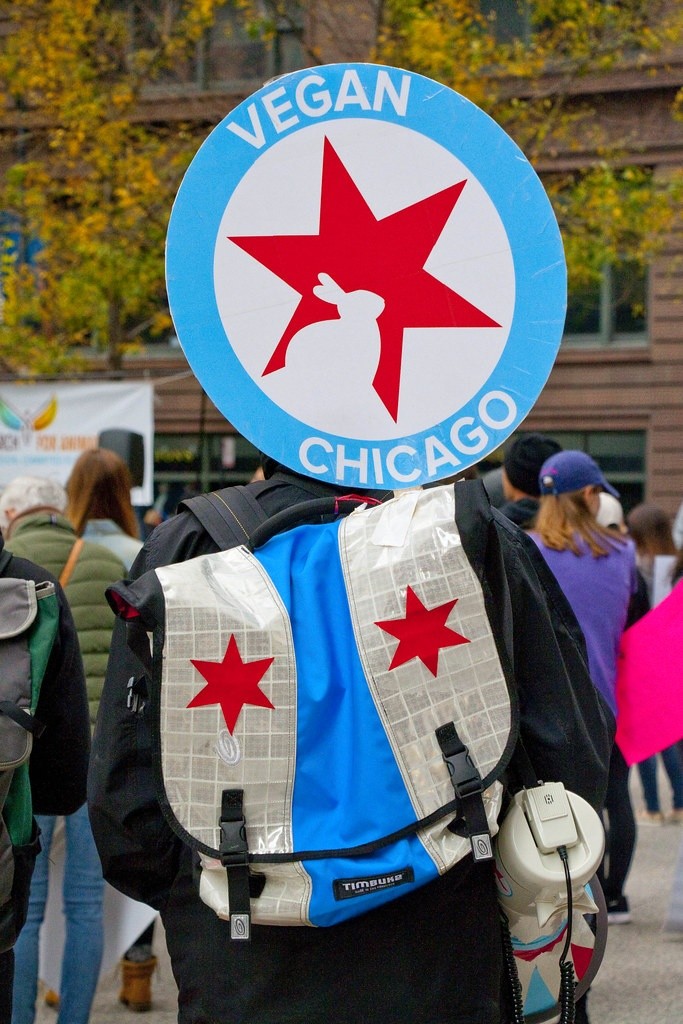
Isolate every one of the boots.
[119,956,156,1010]
[39,980,60,1009]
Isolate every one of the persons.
[1,475,129,1023]
[483,432,564,530]
[522,451,634,924]
[596,493,651,630]
[144,479,189,524]
[99,462,637,1024]
[630,503,683,826]
[0,529,92,1024]
[45,449,157,1010]
[669,503,683,595]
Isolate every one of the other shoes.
[664,809,683,821]
[635,808,664,827]
[607,896,629,924]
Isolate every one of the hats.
[504,434,564,495]
[539,450,620,498]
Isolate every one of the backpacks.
[104,480,516,943]
[0,552,44,955]
